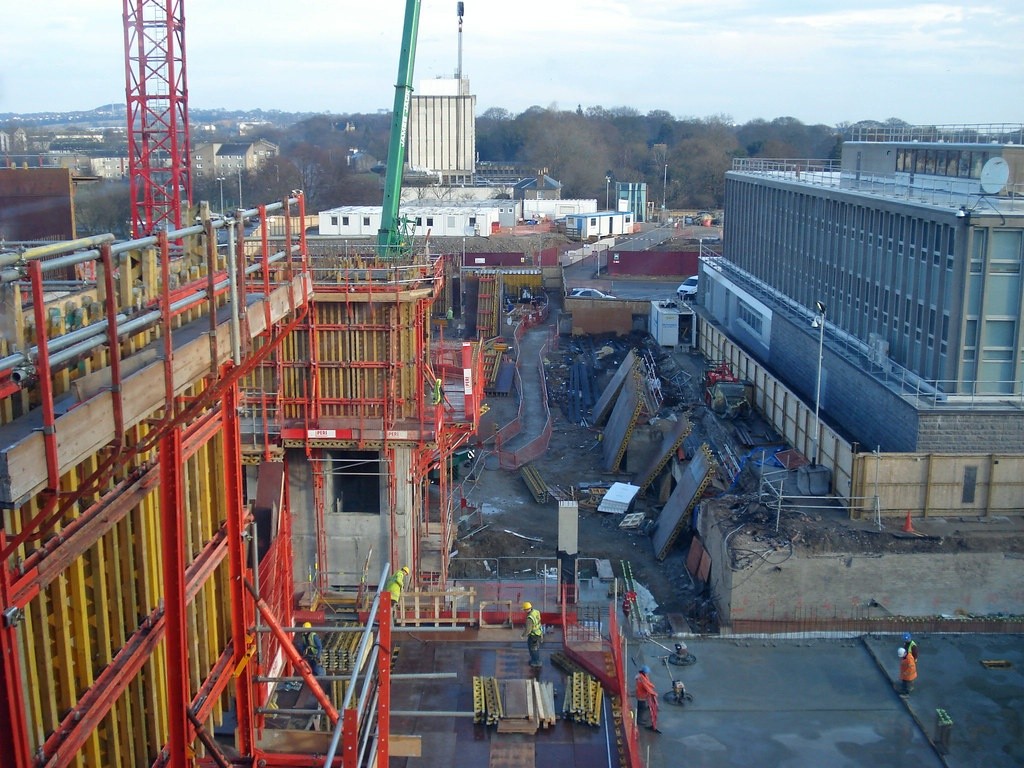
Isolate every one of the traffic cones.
[902,511,914,531]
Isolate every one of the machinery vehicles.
[429,444,476,484]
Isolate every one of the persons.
[635,663,659,730]
[522,602,543,667]
[297,622,326,677]
[621,594,631,624]
[897,632,919,699]
[386,567,410,607]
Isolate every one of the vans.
[677,275,700,301]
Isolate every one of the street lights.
[158,184,168,234]
[605,175,612,212]
[810,300,827,470]
[663,164,668,207]
[274,164,280,183]
[236,163,242,208]
[217,177,227,219]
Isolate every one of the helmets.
[903,633,911,640]
[521,602,532,610]
[898,647,905,657]
[303,622,311,628]
[402,567,409,575]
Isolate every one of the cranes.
[375,0,465,266]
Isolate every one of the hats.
[642,666,649,673]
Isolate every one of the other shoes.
[531,662,542,667]
[899,694,910,699]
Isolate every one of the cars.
[570,288,616,299]
